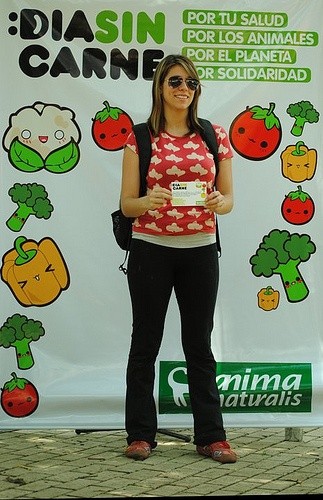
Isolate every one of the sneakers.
[197,440,237,463]
[126,440,152,460]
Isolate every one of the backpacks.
[111,116,220,252]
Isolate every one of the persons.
[120,55,239,465]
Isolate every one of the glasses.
[162,75,202,90]
